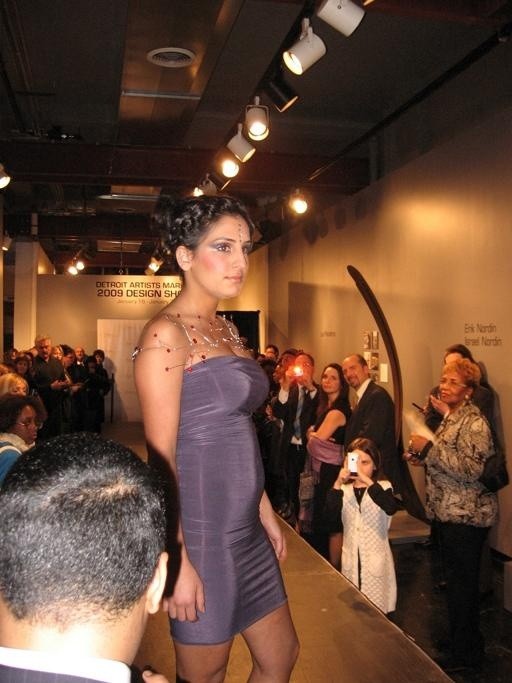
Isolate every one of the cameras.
[289,366,303,377]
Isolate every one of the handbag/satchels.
[478,454,511,493]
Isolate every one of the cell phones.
[347,453,358,473]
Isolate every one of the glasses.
[17,421,44,429]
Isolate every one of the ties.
[293,387,309,440]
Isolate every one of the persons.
[2,432,173,683]
[1,332,116,486]
[328,437,401,617]
[416,341,506,683]
[130,190,303,683]
[236,334,394,550]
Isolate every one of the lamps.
[141,0,371,277]
[2,237,13,251]
[65,242,96,279]
[0,162,12,188]
